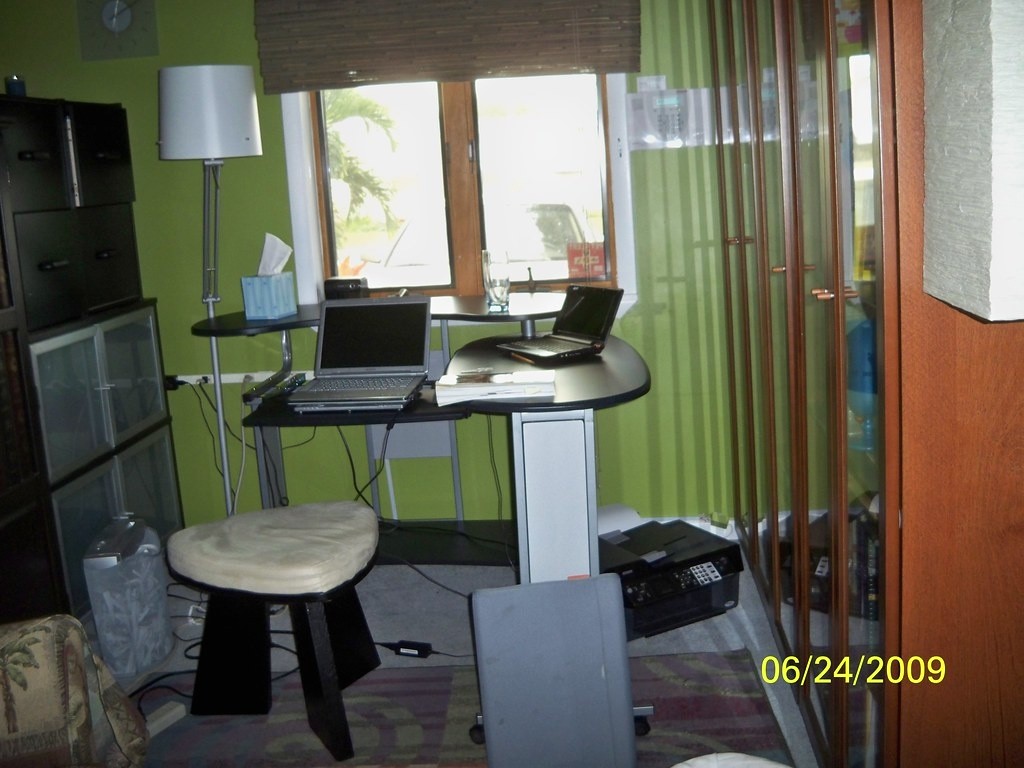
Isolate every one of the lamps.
[159,65,265,516]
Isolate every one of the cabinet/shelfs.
[0,93,185,629]
[708,1,1024,768]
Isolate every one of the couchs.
[0,615,154,768]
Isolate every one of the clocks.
[76,0,159,61]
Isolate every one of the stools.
[167,500,382,760]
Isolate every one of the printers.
[598,519,743,643]
[775,489,879,621]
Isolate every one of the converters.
[395,640,432,658]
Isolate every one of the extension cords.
[144,701,187,738]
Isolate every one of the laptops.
[286,294,431,405]
[496,284,624,362]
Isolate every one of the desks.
[191,292,652,585]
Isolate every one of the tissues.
[237,231,299,320]
